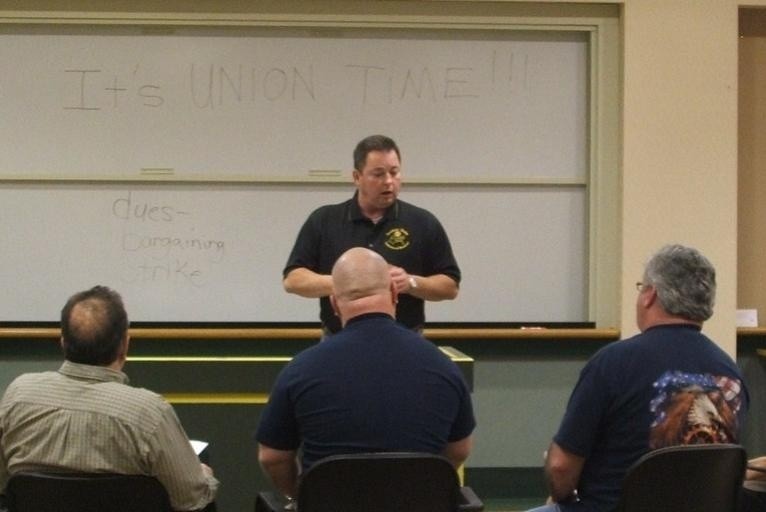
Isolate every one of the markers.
[520,326,547,330]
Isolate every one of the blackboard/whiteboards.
[0,9,621,340]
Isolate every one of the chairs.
[256,452,484,511]
[0,470,217,512]
[617,443,748,512]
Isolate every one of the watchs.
[406,273,417,292]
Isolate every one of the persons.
[250,245,479,503]
[277,133,465,344]
[510,241,751,512]
[0,283,221,510]
